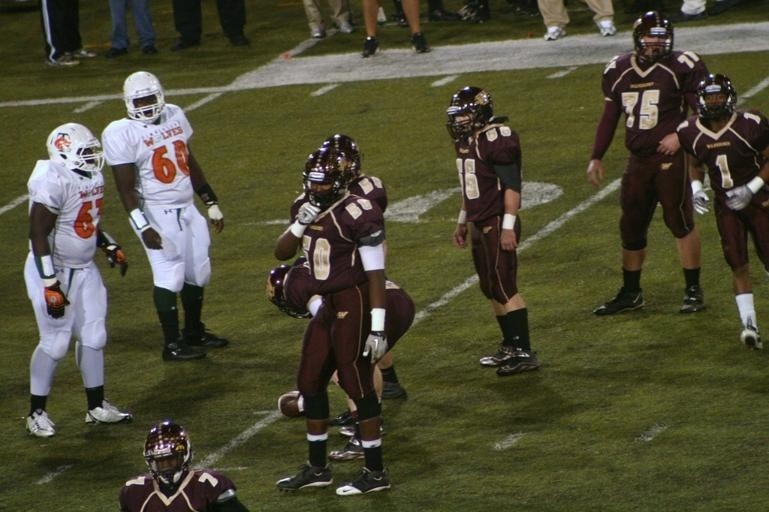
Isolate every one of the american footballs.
[278,391,306,417]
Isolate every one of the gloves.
[43,278,70,319]
[692,189,709,215]
[726,185,752,210]
[106,244,128,278]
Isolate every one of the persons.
[119,421,249,512]
[170,0,246,51]
[103,0,158,57]
[40,0,97,66]
[23,123,132,436]
[102,71,228,360]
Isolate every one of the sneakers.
[593,289,644,316]
[410,33,429,53]
[328,382,406,460]
[276,461,333,494]
[84,398,133,425]
[597,21,618,37]
[182,332,228,347]
[497,348,539,374]
[162,341,205,360]
[544,27,567,40]
[48,31,250,66]
[334,467,392,498]
[680,286,706,313]
[361,36,380,61]
[26,410,57,439]
[670,11,709,22]
[308,5,539,38]
[480,345,515,367]
[741,324,762,349]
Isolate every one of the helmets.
[143,421,192,494]
[124,70,166,124]
[265,265,291,310]
[633,10,674,59]
[695,71,736,118]
[322,134,361,181]
[445,86,494,142]
[47,122,107,193]
[302,147,345,210]
